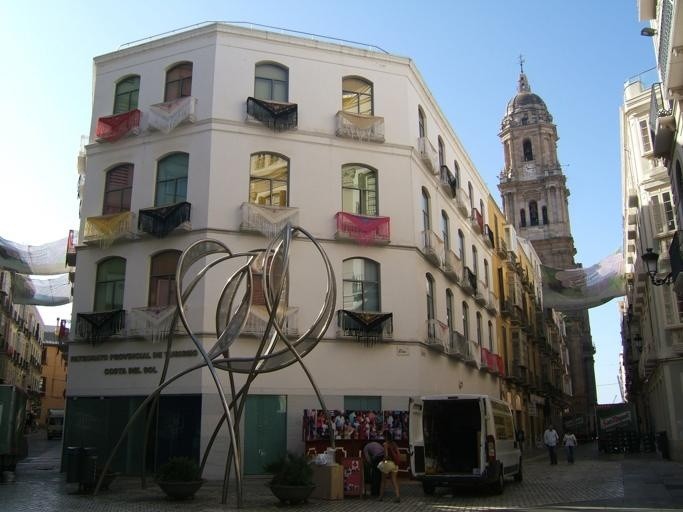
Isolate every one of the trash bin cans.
[656,432,669,459]
[76,442,109,495]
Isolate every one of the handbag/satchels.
[377,457,397,476]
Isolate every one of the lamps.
[632,333,643,354]
[640,246,677,286]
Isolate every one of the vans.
[403,393,524,495]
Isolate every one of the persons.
[303,409,409,441]
[375,429,401,503]
[562,428,578,464]
[542,423,559,465]
[361,437,385,498]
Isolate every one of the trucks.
[43,407,63,441]
[0,384,29,485]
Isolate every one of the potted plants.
[263,450,317,506]
[155,456,209,499]
